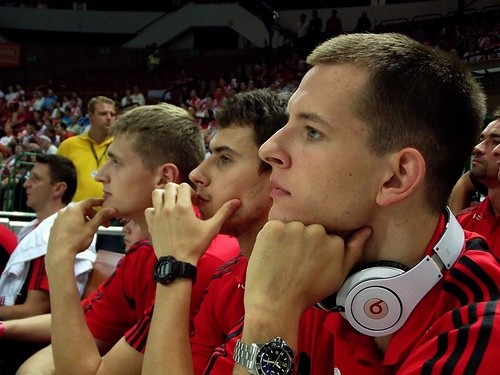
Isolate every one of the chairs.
[27,56,223,115]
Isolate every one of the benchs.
[0,218,126,300]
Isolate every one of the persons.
[141,88,294,375]
[0,8,500,216]
[456,114,500,259]
[0,153,98,320]
[232,32,500,375]
[0,101,242,375]
[56,96,125,254]
[122,215,151,253]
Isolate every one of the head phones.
[313,205,466,337]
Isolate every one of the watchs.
[154,255,199,286]
[233,339,296,375]
